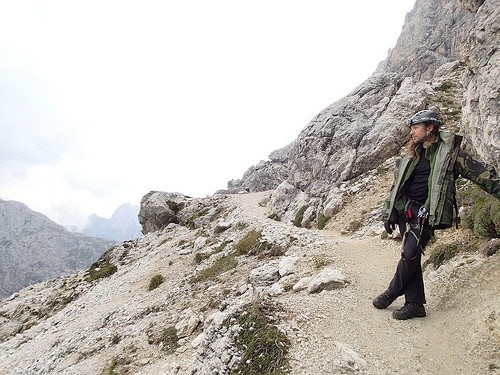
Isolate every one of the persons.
[373,109,500,321]
